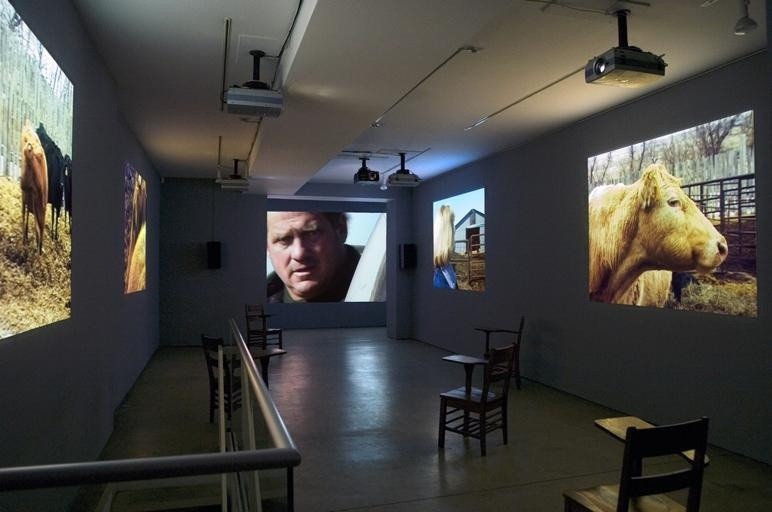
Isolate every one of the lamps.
[214,134,223,184]
[734,0,757,35]
[379,172,388,191]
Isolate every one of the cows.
[18,121,76,256]
[589,163,728,305]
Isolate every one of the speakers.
[206,241,222,269]
[399,243,417,270]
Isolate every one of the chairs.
[474,316,525,390]
[561,414,713,512]
[437,342,517,457]
[201,331,288,425]
[244,302,283,350]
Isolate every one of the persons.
[432,203,459,290]
[266,210,361,304]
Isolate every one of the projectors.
[225,88,283,117]
[220,178,250,192]
[584,48,665,88]
[387,174,420,188]
[353,170,379,183]
